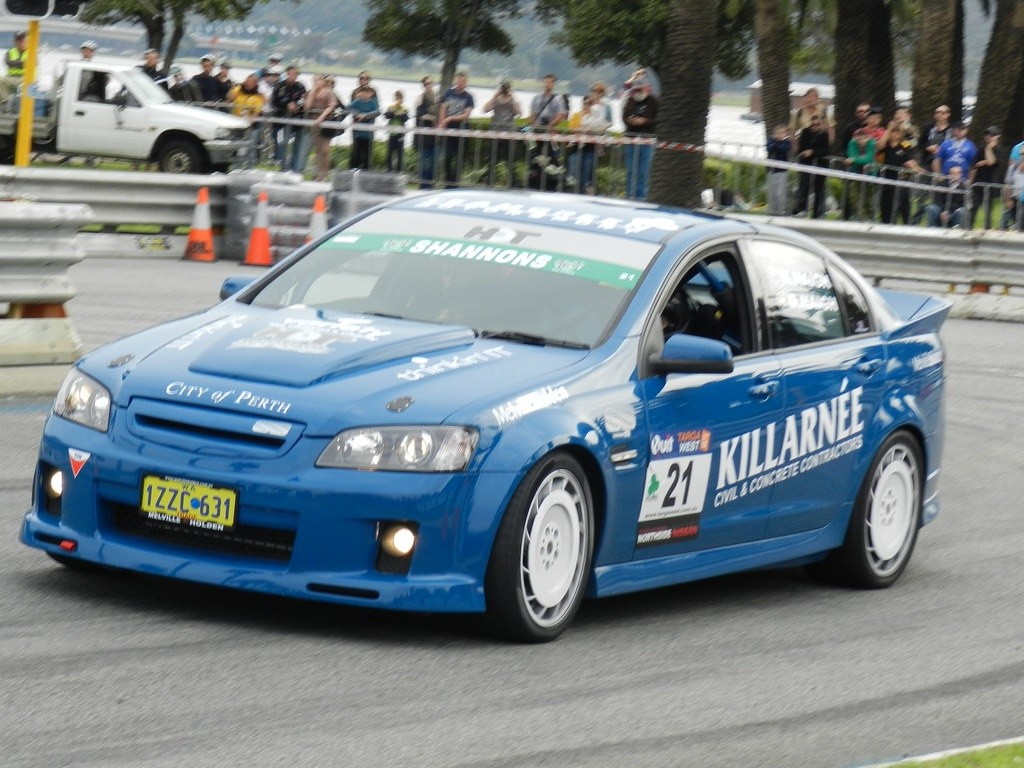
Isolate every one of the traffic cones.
[177,187,221,263]
[238,192,275,268]
[303,194,330,246]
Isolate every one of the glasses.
[592,89,604,93]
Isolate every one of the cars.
[13,186,957,648]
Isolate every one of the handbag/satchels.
[321,115,345,138]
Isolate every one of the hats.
[982,126,1001,134]
[268,53,284,61]
[13,30,26,40]
[80,41,96,50]
[199,54,216,66]
[168,66,182,75]
[267,67,285,73]
[624,68,649,86]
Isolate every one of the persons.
[661,283,699,343]
[766,122,792,215]
[1002,141,1024,231]
[80,40,97,59]
[570,81,613,194]
[349,70,381,170]
[791,87,835,219]
[969,126,1004,229]
[924,166,970,229]
[412,72,474,191]
[839,101,923,225]
[168,53,338,182]
[622,78,657,199]
[484,78,521,164]
[6,31,28,77]
[530,73,567,191]
[138,50,168,89]
[917,104,952,210]
[385,91,410,172]
[932,121,979,184]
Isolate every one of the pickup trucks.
[0,51,253,177]
